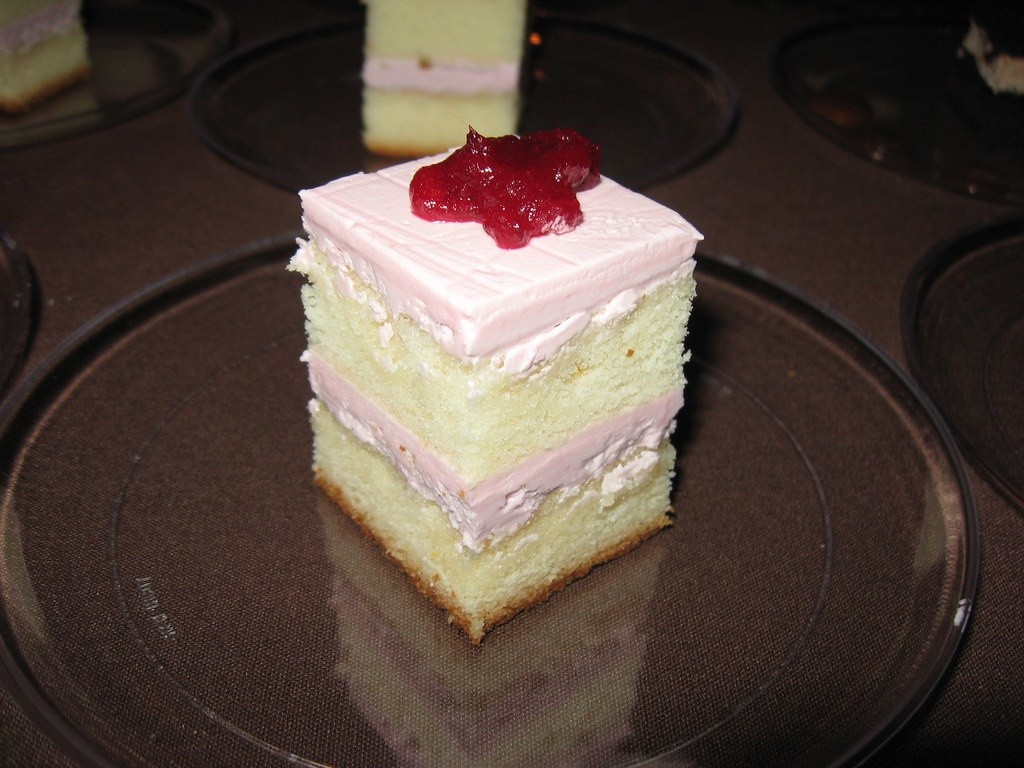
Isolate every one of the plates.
[186,12,743,195]
[0,0,231,151]
[0,229,33,391]
[899,212,1024,515]
[775,17,1024,204]
[0,233,980,768]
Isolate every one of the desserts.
[360,0,527,160]
[0,0,94,120]
[284,126,706,648]
